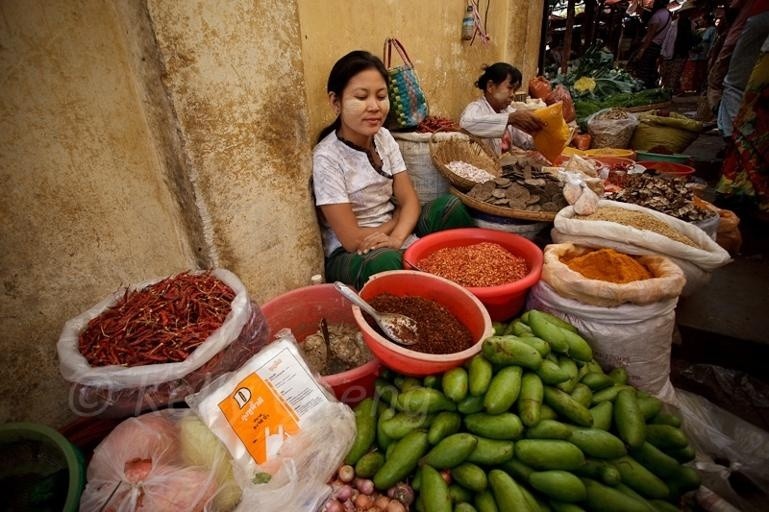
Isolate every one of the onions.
[322,465,451,512]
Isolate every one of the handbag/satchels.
[385,37,428,126]
[629,47,645,63]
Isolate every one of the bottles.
[460,5,476,42]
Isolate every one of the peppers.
[399,114,455,133]
[79,267,266,403]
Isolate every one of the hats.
[676,3,697,13]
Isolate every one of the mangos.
[343,308,702,512]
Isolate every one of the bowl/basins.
[353,270,493,379]
[403,227,545,323]
[587,149,695,179]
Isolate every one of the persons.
[313,51,469,292]
[571,0,768,258]
[459,63,548,158]
